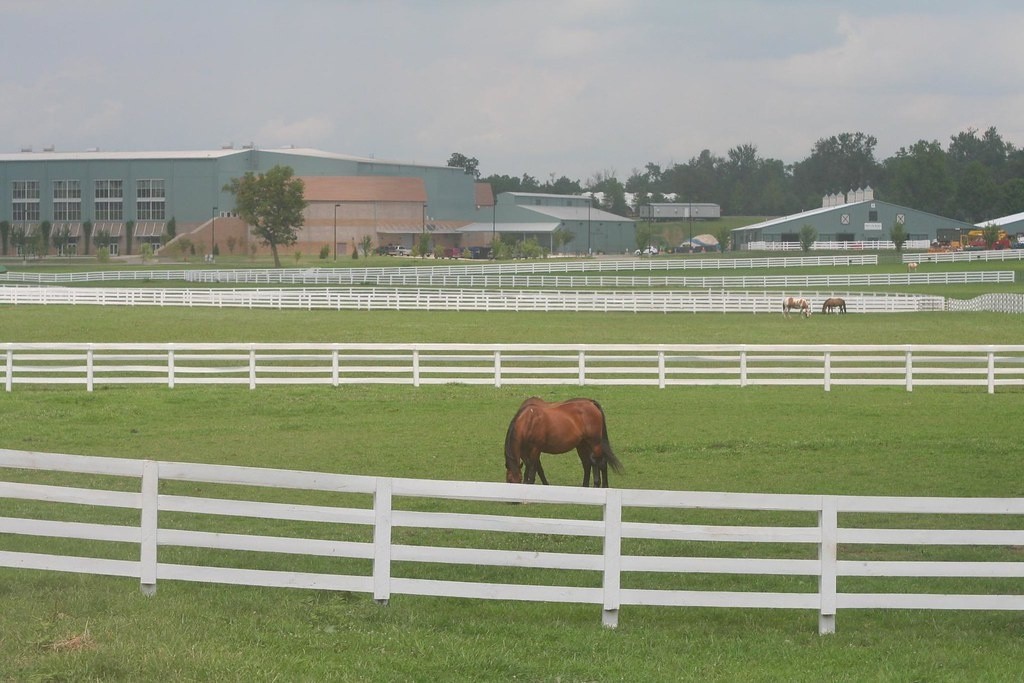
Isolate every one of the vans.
[434,247,460,260]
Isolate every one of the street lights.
[492,199,498,238]
[421,203,428,237]
[212,205,219,258]
[333,203,341,263]
[582,200,592,251]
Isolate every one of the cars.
[388,245,412,256]
[634,246,658,255]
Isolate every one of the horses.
[783,297,847,319]
[907,263,920,272]
[505,396,627,489]
